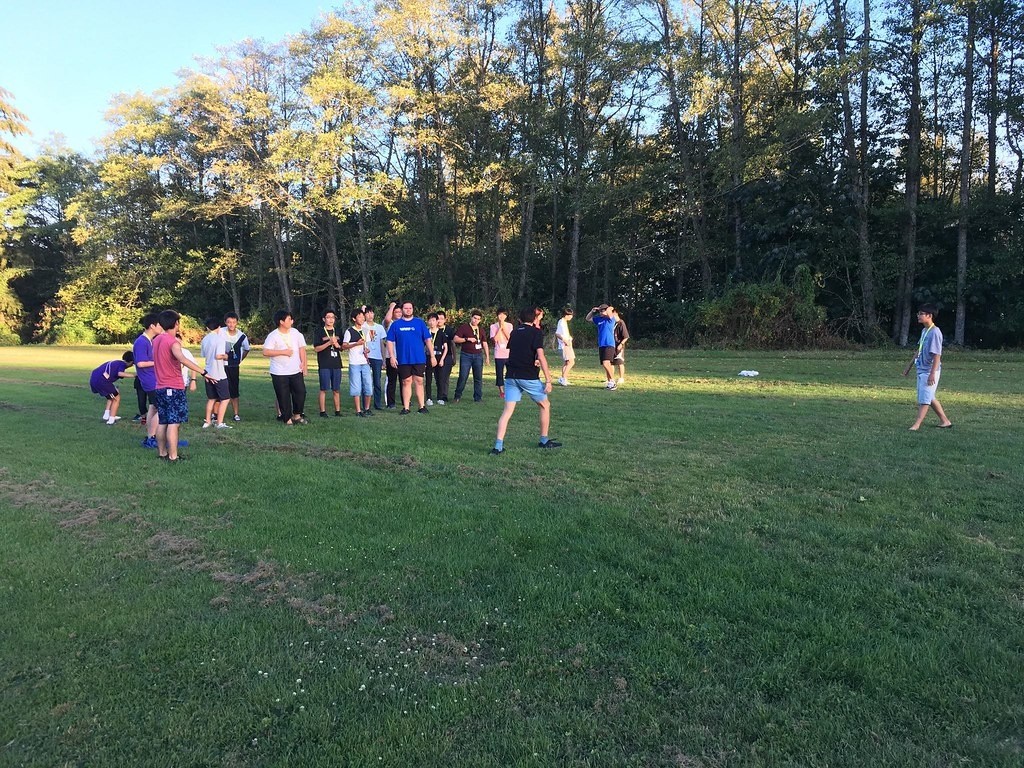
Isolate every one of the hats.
[598,304,609,310]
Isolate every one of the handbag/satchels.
[562,344,576,361]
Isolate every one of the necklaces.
[143,332,152,346]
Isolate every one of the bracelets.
[191,378,197,381]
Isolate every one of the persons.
[903,303,953,431]
[89,350,138,426]
[555,308,576,386]
[489,306,562,455]
[386,300,437,415]
[313,311,345,417]
[586,304,630,391]
[454,309,491,403]
[342,308,376,418]
[130,312,164,450]
[200,316,235,431]
[204,310,250,423]
[362,302,388,411]
[424,310,456,406]
[381,303,413,410]
[152,309,219,462]
[263,310,312,425]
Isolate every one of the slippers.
[936,423,953,429]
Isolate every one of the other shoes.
[102,410,241,464]
[356,408,374,416]
[277,411,311,425]
[399,408,411,415]
[409,401,413,406]
[499,392,506,398]
[538,439,562,448]
[451,398,460,403]
[606,382,618,391]
[488,448,506,455]
[437,399,445,405]
[320,411,344,418]
[618,378,624,383]
[475,399,483,403]
[558,377,570,386]
[417,406,429,414]
[426,399,434,406]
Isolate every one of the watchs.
[465,337,468,341]
[201,369,208,376]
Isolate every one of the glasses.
[917,312,928,315]
[325,315,336,319]
